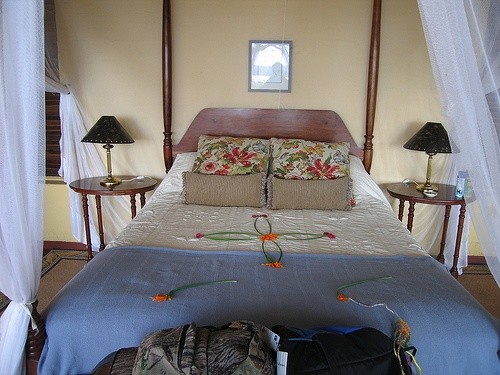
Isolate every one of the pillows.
[270,137,352,179]
[182,171,267,209]
[266,174,355,212]
[191,135,272,175]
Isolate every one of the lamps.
[80,115,135,189]
[403,121,460,198]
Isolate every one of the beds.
[0,106,500,375]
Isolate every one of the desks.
[68,176,157,264]
[386,182,477,281]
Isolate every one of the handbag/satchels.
[270,322,419,375]
[130,318,279,375]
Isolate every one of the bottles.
[455,170,473,201]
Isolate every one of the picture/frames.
[248,39,293,94]
